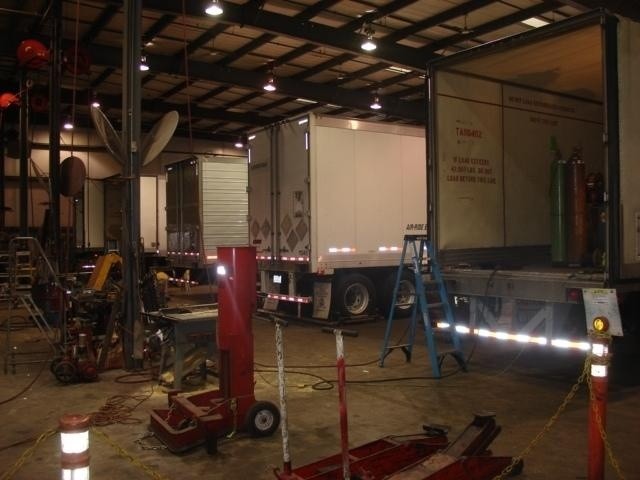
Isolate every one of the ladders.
[378,234,468,380]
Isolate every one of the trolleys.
[48,252,124,384]
[146,243,281,454]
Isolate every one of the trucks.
[243,110,427,326]
[70,153,248,289]
[420,9,638,374]
